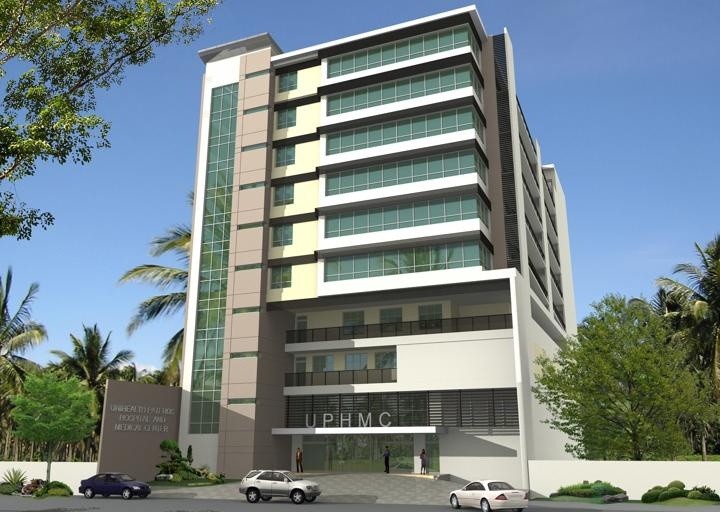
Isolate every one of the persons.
[419,447,427,475]
[384,445,391,474]
[295,446,304,473]
